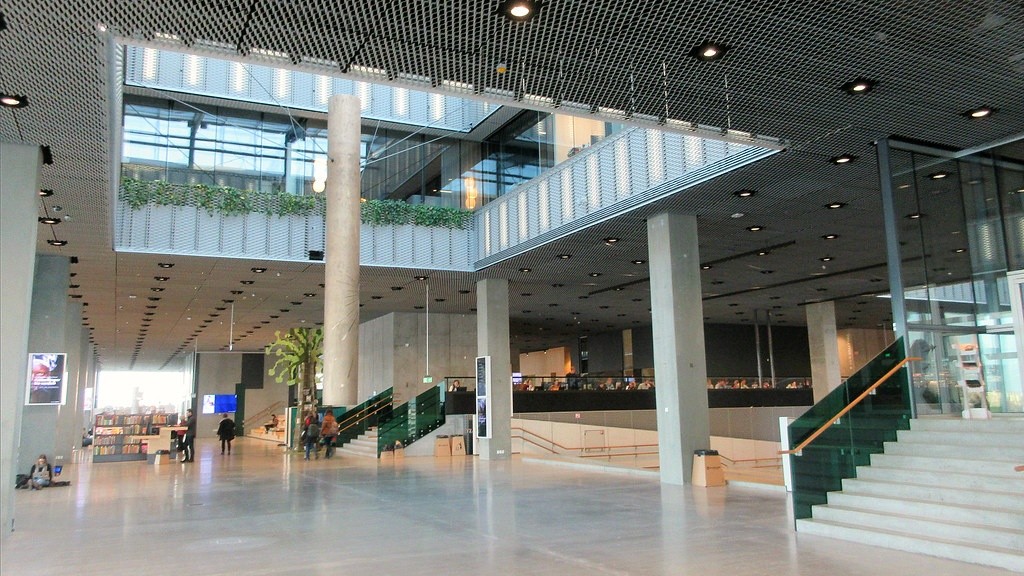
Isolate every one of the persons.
[450,380,460,392]
[786,381,810,388]
[708,379,749,389]
[566,366,578,389]
[28,455,52,491]
[180,409,196,463]
[175,415,186,451]
[264,414,277,434]
[301,409,336,460]
[597,378,651,390]
[216,413,235,455]
[519,376,559,390]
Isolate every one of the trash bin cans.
[154,450,170,464]
[691,450,725,488]
[436,434,467,456]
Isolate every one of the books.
[94,416,166,455]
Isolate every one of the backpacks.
[308,423,318,437]
[331,421,339,434]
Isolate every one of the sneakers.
[228,452,230,455]
[187,459,194,462]
[221,452,224,455]
[180,459,189,462]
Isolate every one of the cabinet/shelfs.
[955,339,991,419]
[92,414,178,464]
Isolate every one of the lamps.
[464,177,477,210]
[313,157,327,193]
